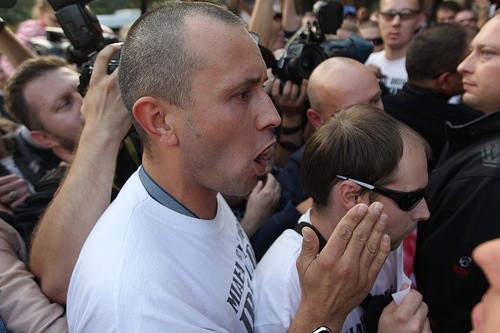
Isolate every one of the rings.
[9,190,18,200]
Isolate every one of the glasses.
[336,173,428,212]
[378,7,421,22]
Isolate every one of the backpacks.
[276,25,330,96]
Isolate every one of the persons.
[0,0,500,333]
[66,2,392,333]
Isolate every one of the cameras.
[248,30,328,93]
[47,0,122,75]
